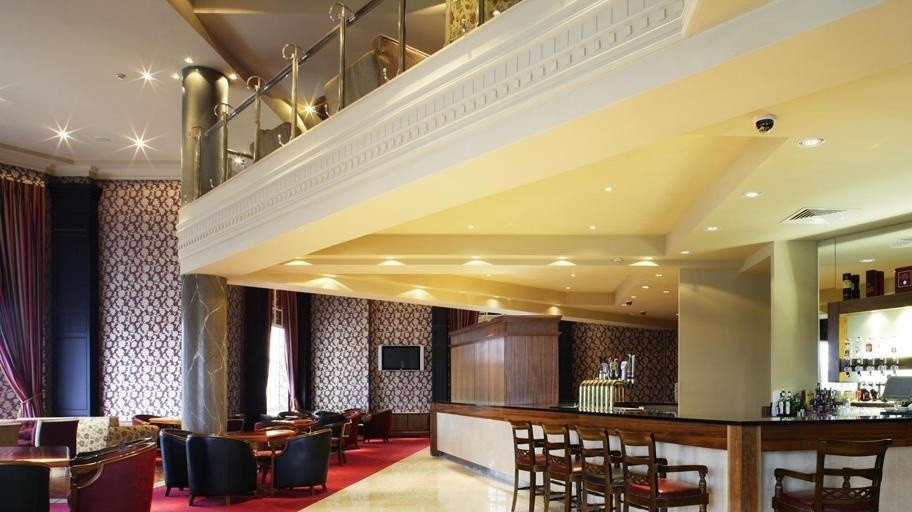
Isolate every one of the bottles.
[614,358,619,378]
[775,382,833,418]
[843,333,902,372]
[876,271,884,295]
[866,270,878,297]
[850,274,859,299]
[598,370,606,380]
[841,272,852,300]
[609,363,616,379]
[859,384,877,401]
[621,361,628,381]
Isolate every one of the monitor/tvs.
[882,376,912,407]
[378,345,424,372]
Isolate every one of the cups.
[894,400,905,410]
[836,403,886,416]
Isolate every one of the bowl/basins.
[897,407,908,413]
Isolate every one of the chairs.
[768,435,891,512]
[614,426,709,512]
[572,423,626,511]
[505,418,562,511]
[540,422,584,511]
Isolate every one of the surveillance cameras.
[753,114,776,134]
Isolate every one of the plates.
[885,408,896,412]
